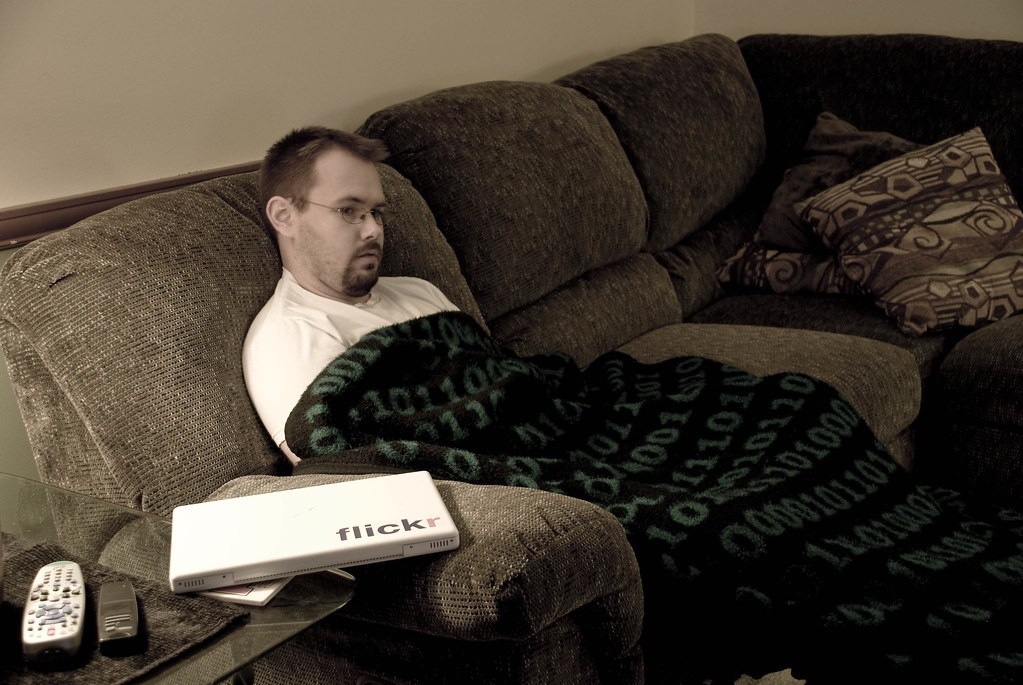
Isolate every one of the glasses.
[285,196,393,225]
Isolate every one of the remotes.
[21,561,86,659]
[97,581,139,650]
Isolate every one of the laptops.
[169,470,460,593]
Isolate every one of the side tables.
[0,470,358,685]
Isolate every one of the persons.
[242,127,461,466]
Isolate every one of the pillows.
[714,110,927,300]
[794,127,1023,337]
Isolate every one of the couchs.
[0,31,1023,685]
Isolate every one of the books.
[199,576,296,607]
